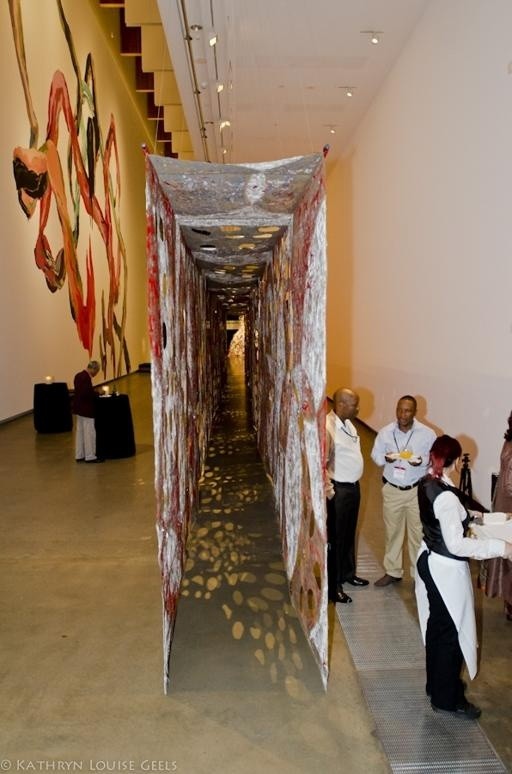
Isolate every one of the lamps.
[337,86,359,100]
[323,124,342,136]
[359,30,385,46]
[189,24,232,158]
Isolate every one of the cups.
[46,376,52,384]
[102,385,109,395]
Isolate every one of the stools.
[138,362,151,374]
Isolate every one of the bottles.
[112,384,117,397]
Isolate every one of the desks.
[32,382,72,436]
[77,392,136,462]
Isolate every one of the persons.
[476,408,512,622]
[74,360,105,463]
[416,435,511,718]
[325,388,369,603]
[370,395,438,587]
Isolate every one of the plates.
[471,514,511,526]
[387,452,420,463]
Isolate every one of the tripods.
[459,453,473,498]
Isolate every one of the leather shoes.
[374,574,402,586]
[328,593,352,603]
[347,577,369,585]
[431,700,481,719]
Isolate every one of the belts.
[329,479,358,487]
[387,480,419,489]
